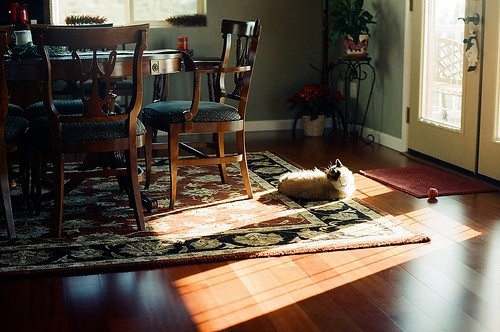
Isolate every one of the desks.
[4,48,184,214]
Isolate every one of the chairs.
[0,19,263,239]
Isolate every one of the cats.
[278,158,356,203]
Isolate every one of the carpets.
[359,166,497,198]
[0,150,430,275]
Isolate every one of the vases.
[301,115,326,136]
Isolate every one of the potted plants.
[325,0,377,58]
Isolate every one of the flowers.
[286,85,345,121]
